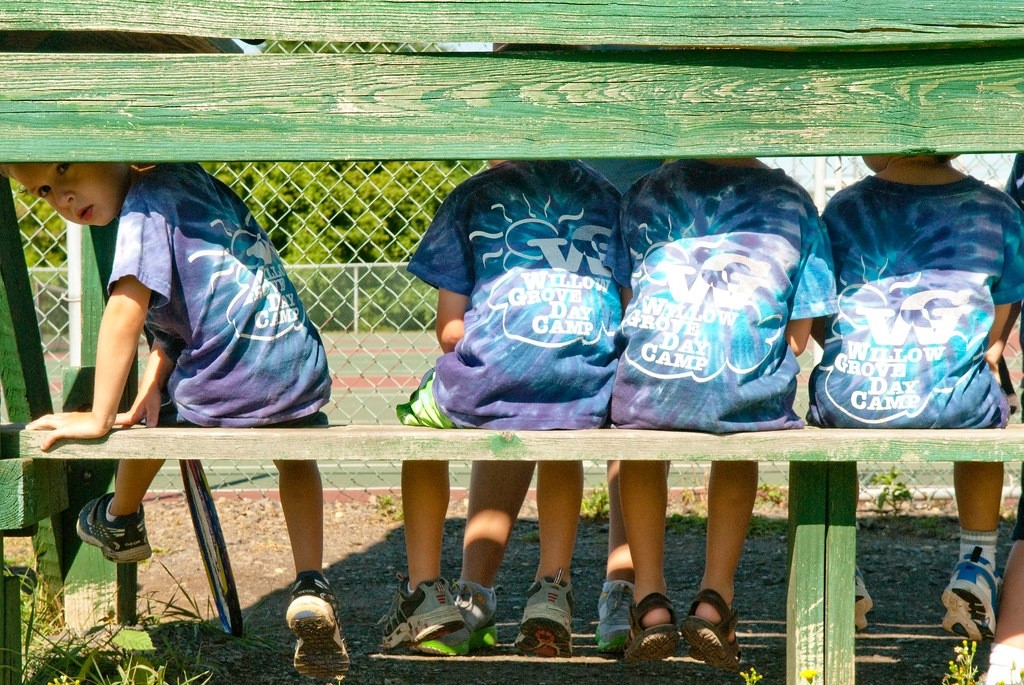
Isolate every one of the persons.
[452,157,668,650]
[807,154,1024,644]
[606,158,839,672]
[987,153,1024,685]
[0,162,350,682]
[367,157,633,657]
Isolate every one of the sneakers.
[514,568,575,656]
[940,546,997,640]
[286,571,349,680]
[595,579,633,651]
[383,570,465,651]
[410,579,504,656]
[855,563,872,631]
[76,492,152,563]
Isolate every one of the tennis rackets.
[142,320,245,638]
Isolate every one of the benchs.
[0,2,1024,685]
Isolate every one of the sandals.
[625,591,678,662]
[681,589,742,670]
